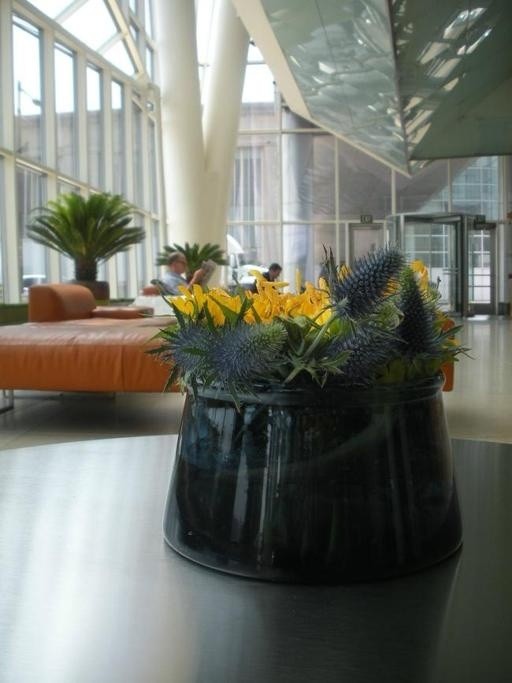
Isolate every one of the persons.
[158,251,205,296]
[250,262,283,293]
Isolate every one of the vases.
[162,396,465,586]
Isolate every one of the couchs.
[0,283,193,413]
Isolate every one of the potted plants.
[21,187,148,300]
